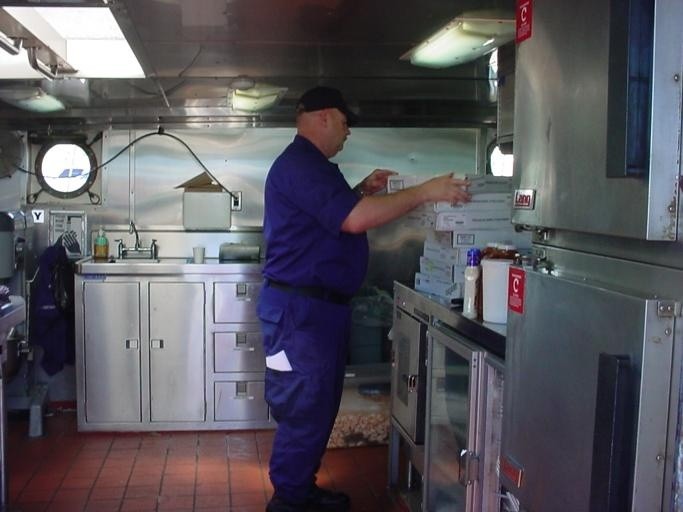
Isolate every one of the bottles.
[94,224,108,259]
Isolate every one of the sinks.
[85,258,190,265]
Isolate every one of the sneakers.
[266,485,349,512]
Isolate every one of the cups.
[192,247,205,263]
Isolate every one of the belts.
[269,281,351,304]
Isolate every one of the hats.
[296,86,357,118]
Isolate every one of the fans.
[0,125,23,180]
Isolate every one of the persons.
[254,83,472,510]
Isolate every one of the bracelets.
[350,184,372,197]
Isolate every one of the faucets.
[128,220,139,248]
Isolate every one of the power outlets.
[230,191,241,210]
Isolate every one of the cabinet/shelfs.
[72,274,212,433]
[212,274,269,431]
